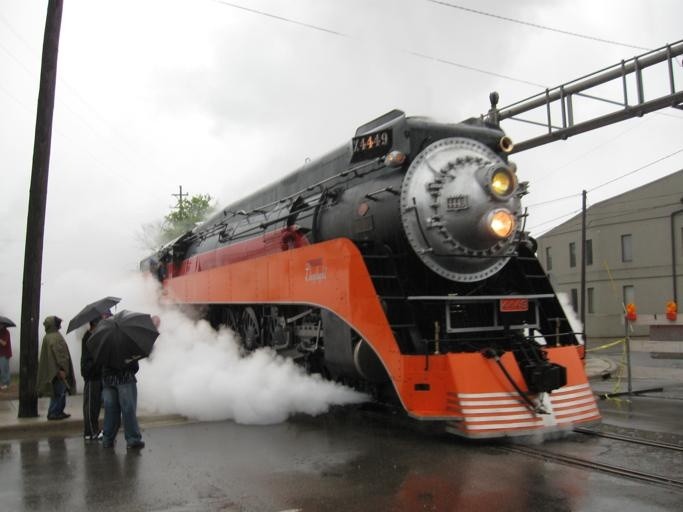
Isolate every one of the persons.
[79,313,102,442]
[34,315,75,421]
[1,325,12,391]
[97,358,145,452]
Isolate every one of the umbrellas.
[85,309,160,368]
[0,315,16,328]
[63,294,122,334]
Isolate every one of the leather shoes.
[126,440,145,450]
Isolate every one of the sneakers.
[83,428,104,441]
[46,412,72,421]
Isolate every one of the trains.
[140,91,603,440]
